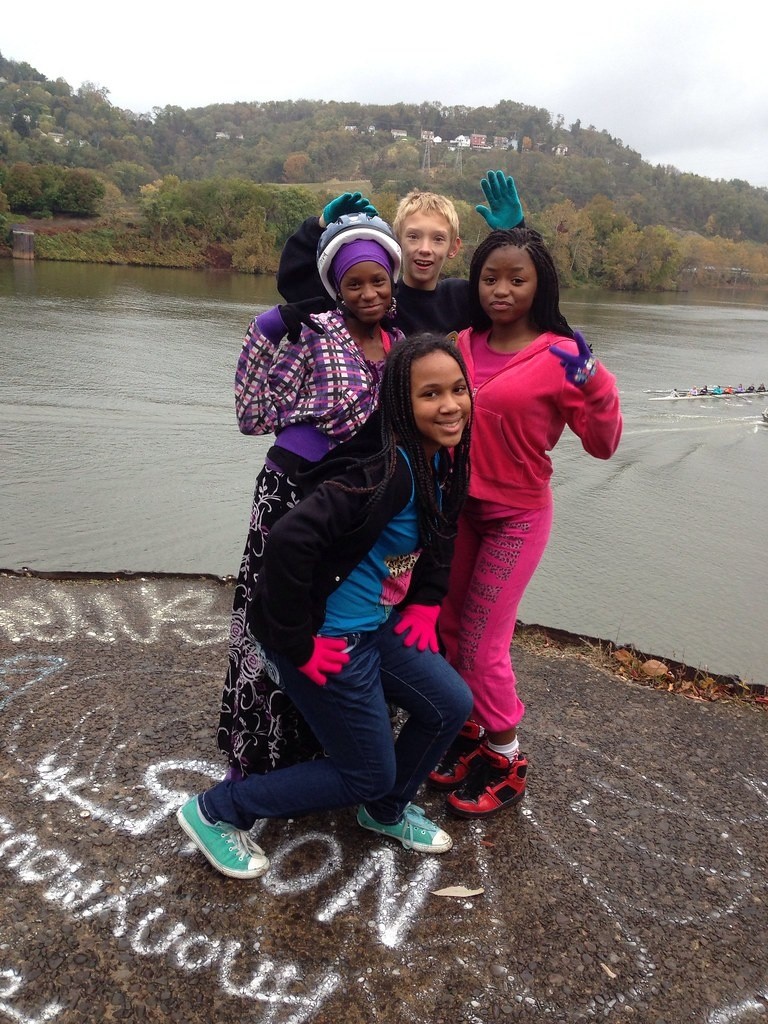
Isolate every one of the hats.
[328,238,393,292]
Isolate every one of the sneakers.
[448,744,528,818]
[175,794,270,879]
[429,721,488,783]
[356,800,453,853]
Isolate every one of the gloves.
[548,331,597,389]
[297,636,350,685]
[476,170,524,231]
[395,603,442,653]
[323,192,379,226]
[278,296,323,345]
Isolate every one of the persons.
[176,327,472,880]
[218,169,628,818]
[669,384,766,396]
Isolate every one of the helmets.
[315,211,402,300]
